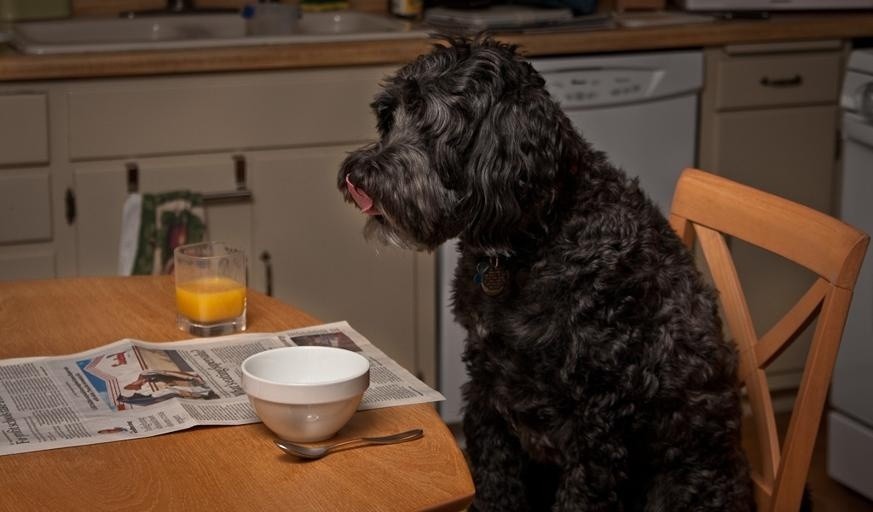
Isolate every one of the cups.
[173,241,246,336]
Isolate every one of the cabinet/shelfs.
[699,40,851,395]
[63,66,418,378]
[0,80,62,274]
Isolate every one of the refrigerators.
[442,55,703,445]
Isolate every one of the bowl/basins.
[240,345,369,442]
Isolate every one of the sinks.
[9,11,434,52]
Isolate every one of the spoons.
[272,427,423,457]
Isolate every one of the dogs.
[335,26,760,511]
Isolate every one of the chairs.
[667,167,871,510]
